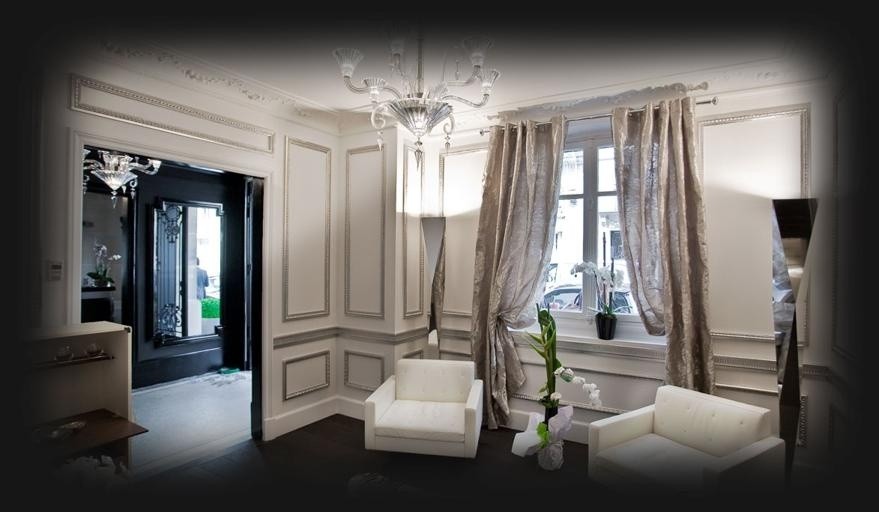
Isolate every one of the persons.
[196,257,209,299]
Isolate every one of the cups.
[82,343,102,357]
[55,344,73,361]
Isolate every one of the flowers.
[569,253,633,317]
[85,237,126,282]
[519,298,602,450]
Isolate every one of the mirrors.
[147,196,229,348]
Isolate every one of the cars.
[541,284,636,317]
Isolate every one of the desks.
[30,318,135,475]
[32,405,150,459]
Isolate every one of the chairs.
[362,358,487,466]
[585,382,787,511]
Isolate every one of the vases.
[593,313,618,341]
[91,276,112,289]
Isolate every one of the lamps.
[330,1,501,167]
[81,145,163,211]
